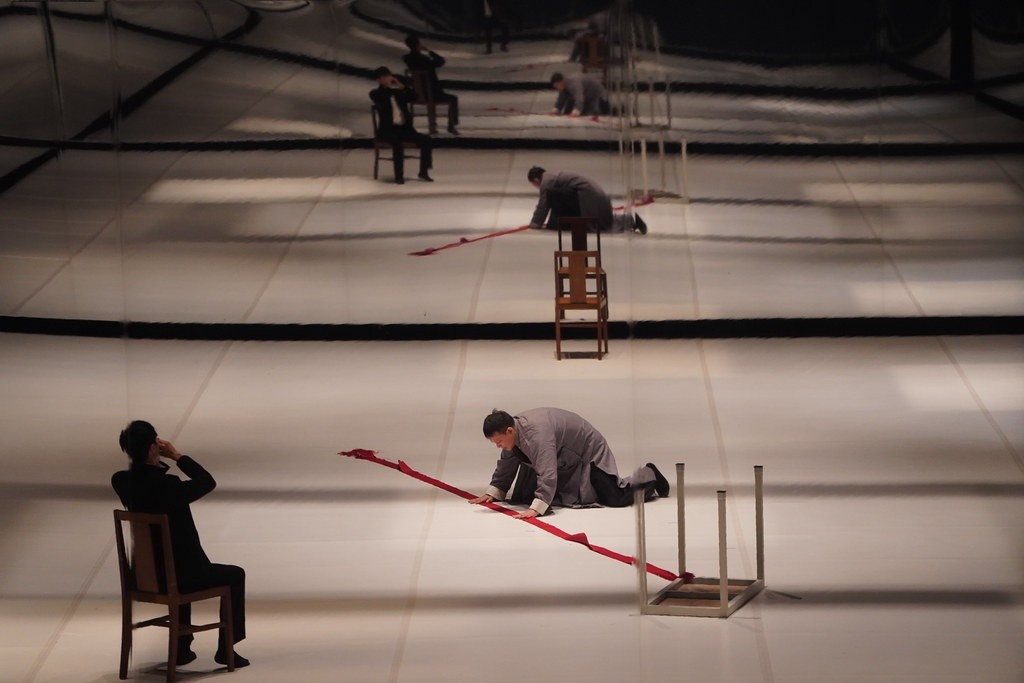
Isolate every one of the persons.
[468,408,670,522]
[370,0,648,235]
[110,419,250,667]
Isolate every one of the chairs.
[554,215,609,360]
[371,102,422,179]
[113,509,235,683]
[404,68,448,120]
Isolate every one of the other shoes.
[418,170,433,182]
[447,126,459,135]
[176,648,197,665]
[215,648,250,668]
[646,462,670,498]
[634,211,647,235]
[396,176,405,184]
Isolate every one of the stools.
[633,462,764,618]
[617,74,689,203]
